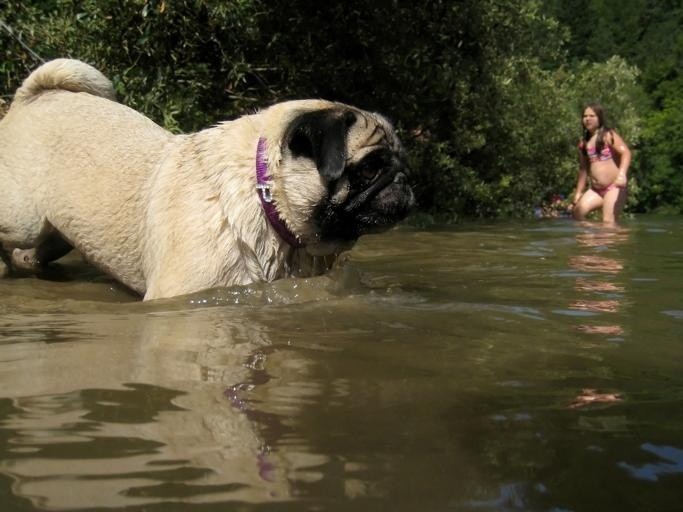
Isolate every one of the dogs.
[0,55,418,306]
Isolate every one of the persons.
[571,104,632,222]
